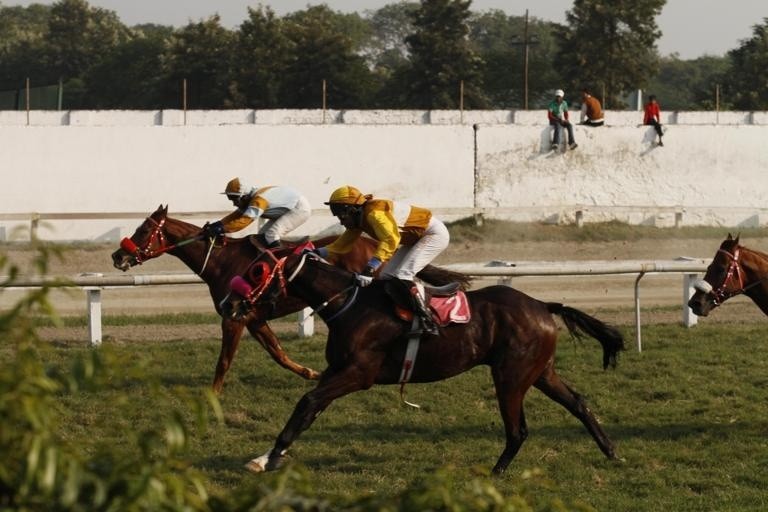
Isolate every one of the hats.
[554,89,565,97]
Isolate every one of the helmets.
[323,186,374,205]
[218,177,248,196]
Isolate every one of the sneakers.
[551,144,558,150]
[570,143,578,150]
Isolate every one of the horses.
[215,257,626,479]
[107,202,476,396]
[685,231,767,319]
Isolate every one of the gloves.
[203,221,224,236]
[352,265,375,288]
[301,248,320,259]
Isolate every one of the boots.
[269,240,282,248]
[402,292,439,338]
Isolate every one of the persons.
[547,88,577,150]
[578,87,604,127]
[296,184,450,339]
[642,93,664,146]
[197,176,311,249]
